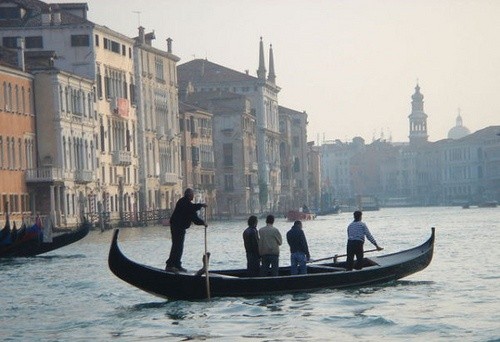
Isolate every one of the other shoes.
[178,267,187,271]
[166,265,178,272]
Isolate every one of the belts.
[291,251,304,253]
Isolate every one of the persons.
[165,188,208,273]
[347,211,382,271]
[259,215,282,276]
[287,221,310,275]
[243,216,260,271]
[299,204,309,212]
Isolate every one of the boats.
[108,225,435,297]
[0,212,91,259]
[287,210,318,222]
